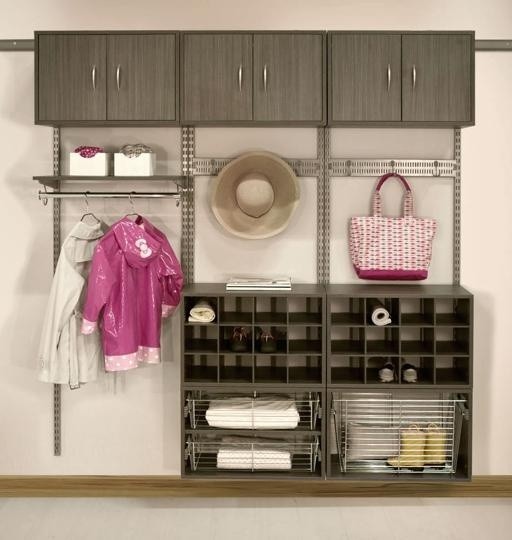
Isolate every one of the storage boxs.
[69,152,112,177]
[114,152,157,177]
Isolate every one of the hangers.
[80,190,100,222]
[126,191,139,219]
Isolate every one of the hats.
[210,151,302,240]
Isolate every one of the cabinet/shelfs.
[325,284,473,481]
[180,283,326,481]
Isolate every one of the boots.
[386,428,426,473]
[424,426,448,470]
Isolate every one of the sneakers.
[232,327,246,352]
[403,368,417,384]
[260,327,278,353]
[379,367,395,383]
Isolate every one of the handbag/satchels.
[349,214,437,281]
